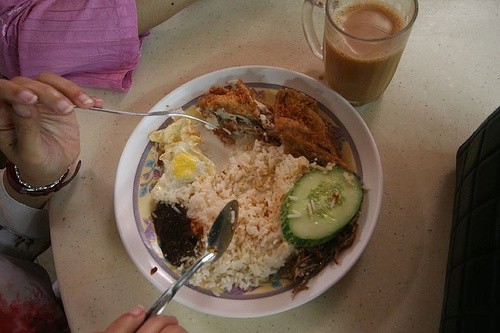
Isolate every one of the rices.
[155,139,356,301]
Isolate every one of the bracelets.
[6,159,81,197]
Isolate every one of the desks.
[48,0,500,333]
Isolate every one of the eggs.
[149,124,216,203]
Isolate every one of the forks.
[33,99,232,136]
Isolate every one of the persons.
[0,0,199,93]
[0,72,187,333]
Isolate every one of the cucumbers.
[280,165,363,248]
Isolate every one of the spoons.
[144,200,238,321]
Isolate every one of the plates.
[113,64,383,319]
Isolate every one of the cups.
[301,0,418,107]
